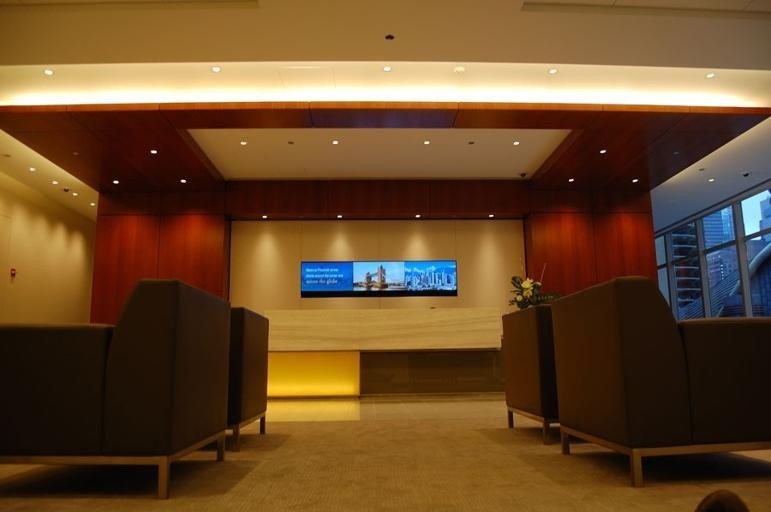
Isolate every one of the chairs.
[501,304,558,444]
[226,305,269,448]
[0,280,231,499]
[551,275,770,488]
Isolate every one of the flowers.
[508,275,554,309]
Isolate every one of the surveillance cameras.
[519,170,528,178]
